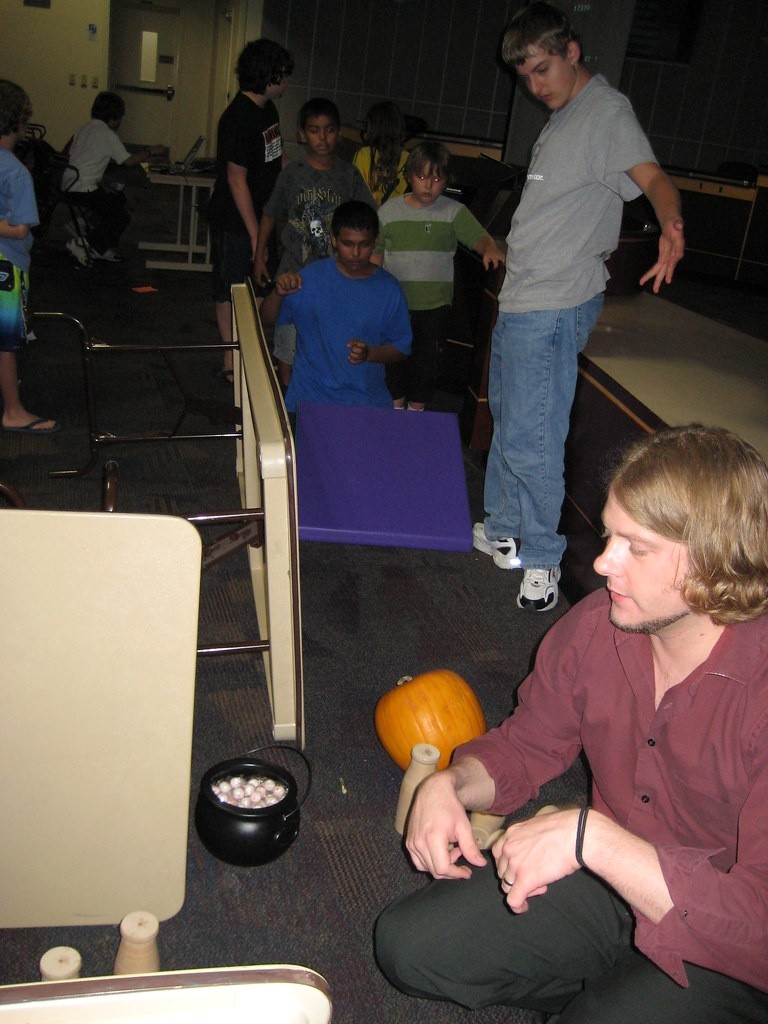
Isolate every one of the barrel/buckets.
[193,740,312,866]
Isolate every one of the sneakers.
[471,522,523,570]
[517,564,561,612]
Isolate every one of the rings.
[503,879,513,886]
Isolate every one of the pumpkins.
[375,670,488,771]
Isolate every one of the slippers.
[1,417,62,434]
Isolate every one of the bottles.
[534,805,559,817]
[395,744,440,835]
[470,812,506,849]
[39,946,83,983]
[114,911,161,975]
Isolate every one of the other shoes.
[220,368,234,386]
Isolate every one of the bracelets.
[576,807,592,866]
[145,150,151,156]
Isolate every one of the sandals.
[90,247,122,261]
[66,238,94,268]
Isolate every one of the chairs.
[14,122,125,273]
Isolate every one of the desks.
[0,460,202,930]
[34,276,307,751]
[137,162,217,272]
[660,162,759,280]
[401,129,504,163]
[460,284,767,593]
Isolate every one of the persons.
[253,98,378,387]
[207,38,296,383]
[474,0,686,612]
[62,92,166,267]
[261,200,413,437]
[369,142,505,410]
[376,426,768,1023]
[352,103,411,205]
[0,79,66,435]
